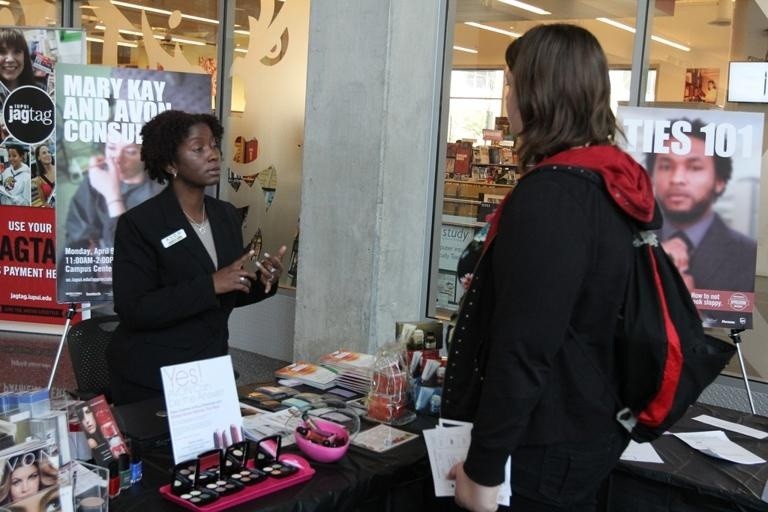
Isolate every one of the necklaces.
[183,203,207,235]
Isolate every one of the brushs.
[295,412,347,449]
[212,423,240,455]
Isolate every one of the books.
[275,348,376,396]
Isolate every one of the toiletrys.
[415,387,435,412]
[399,323,416,345]
[105,442,146,499]
[409,350,423,378]
[420,359,441,383]
[401,322,448,416]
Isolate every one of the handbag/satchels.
[615,229,739,445]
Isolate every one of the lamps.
[451,1,690,55]
[82,3,250,56]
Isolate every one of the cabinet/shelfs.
[434,116,535,313]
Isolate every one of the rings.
[239,277,244,284]
[270,268,276,273]
[268,275,274,281]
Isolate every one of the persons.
[433,21,664,512]
[0,449,58,506]
[0,484,61,511]
[0,146,32,209]
[642,115,760,293]
[77,406,104,447]
[110,111,289,403]
[0,27,55,145]
[35,143,56,207]
[55,115,168,302]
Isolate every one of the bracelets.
[107,198,124,208]
